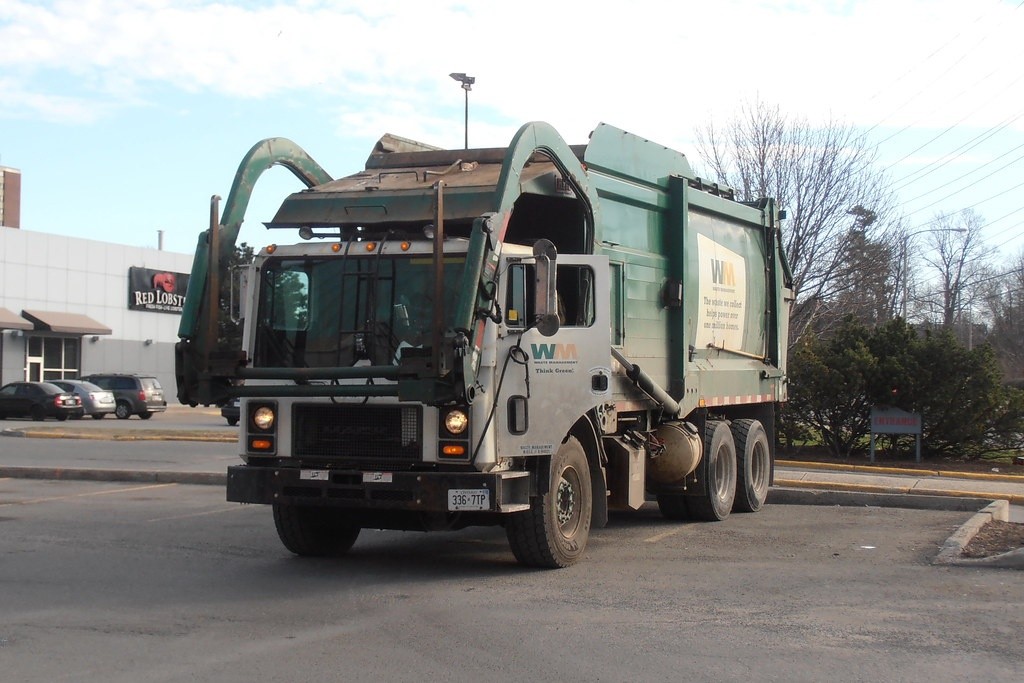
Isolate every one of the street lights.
[450,72,476,148]
[903,228,967,330]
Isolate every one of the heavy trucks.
[174,123,796,568]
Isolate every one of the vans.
[77,373,167,419]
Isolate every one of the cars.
[221,397,240,426]
[44,380,116,419]
[0,382,83,421]
